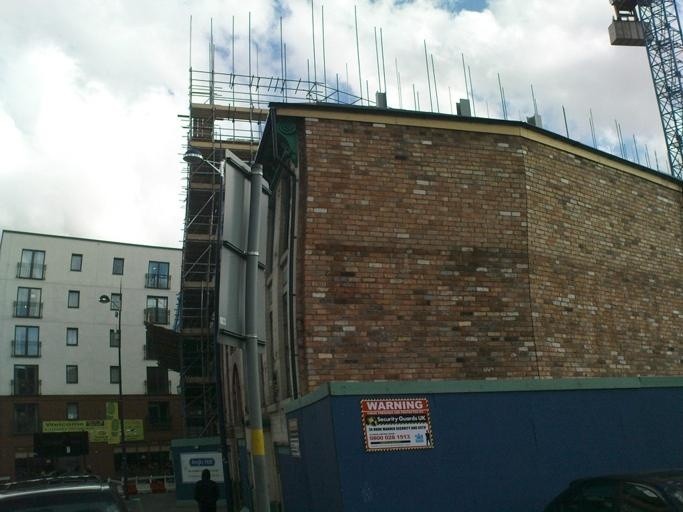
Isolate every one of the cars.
[546,471,682,511]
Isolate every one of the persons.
[194,470,219,512]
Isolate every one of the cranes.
[607,0,683,182]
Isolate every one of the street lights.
[98,276,128,485]
